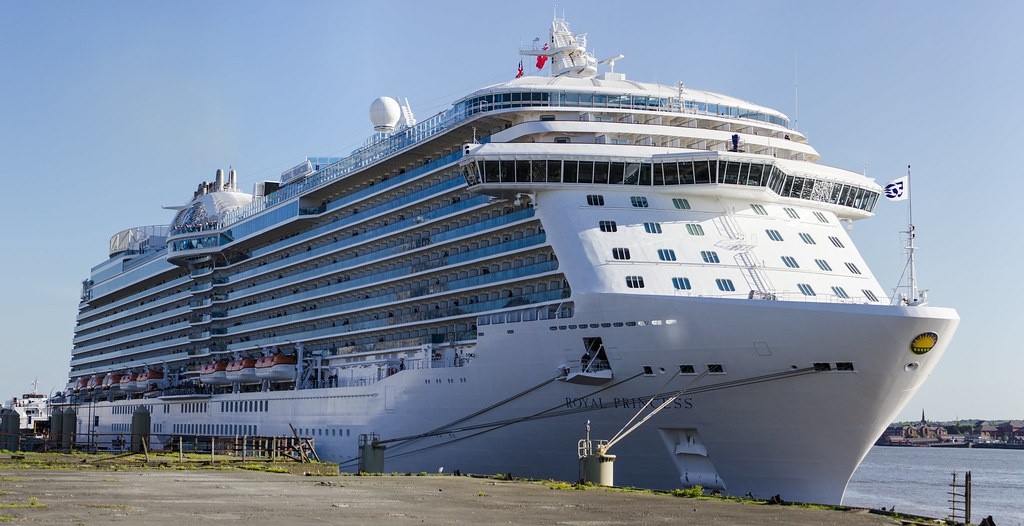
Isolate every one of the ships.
[45,5,961,506]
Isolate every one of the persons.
[581,351,592,373]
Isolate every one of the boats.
[7,377,54,438]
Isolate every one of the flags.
[515,59,524,79]
[883,175,909,202]
[535,42,549,71]
